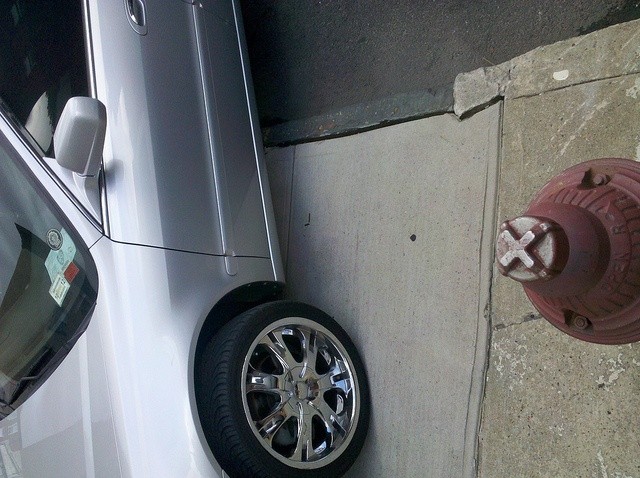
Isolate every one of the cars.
[1,0,370,478]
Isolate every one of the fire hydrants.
[496,159,639,345]
